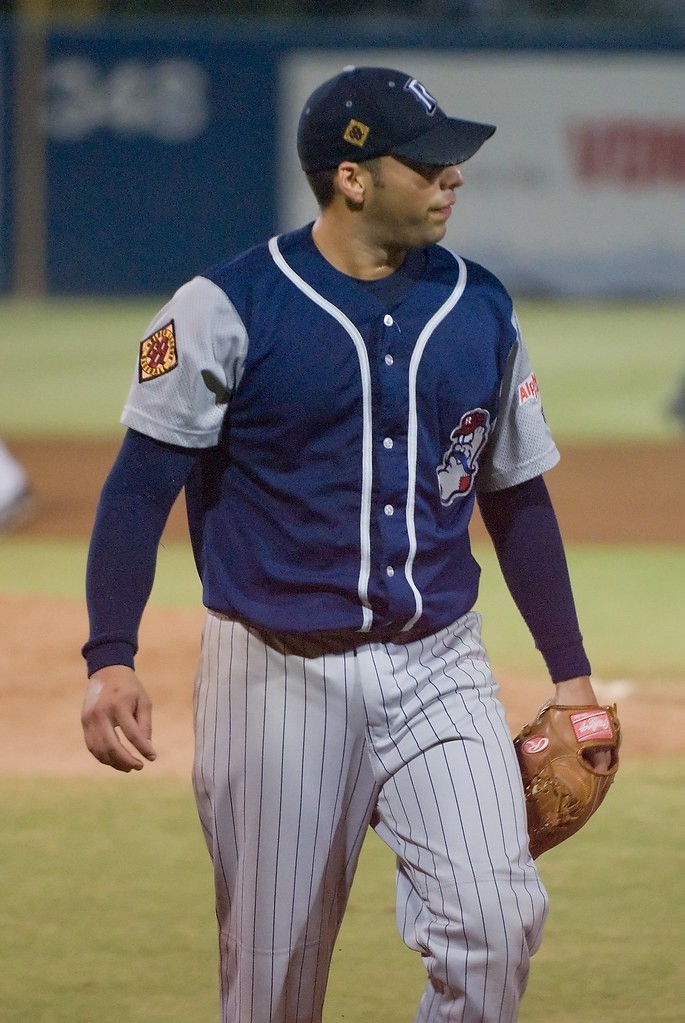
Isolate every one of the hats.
[294,65,498,172]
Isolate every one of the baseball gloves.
[512,701,622,861]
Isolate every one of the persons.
[80,65,623,1023]
